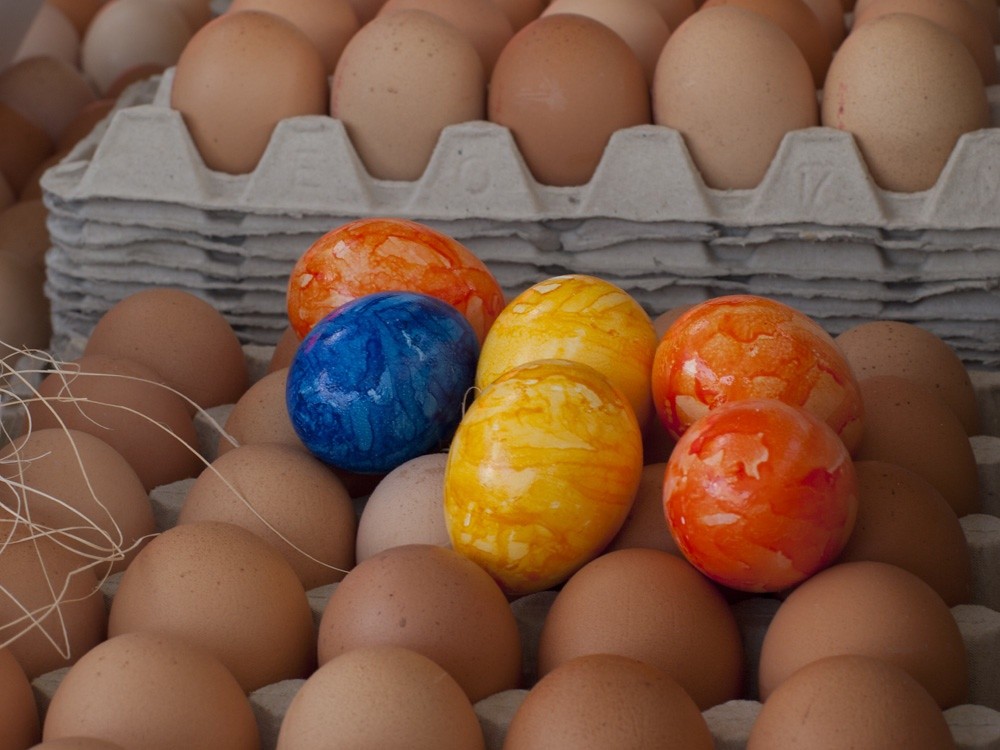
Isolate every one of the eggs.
[0,0,999,750]
